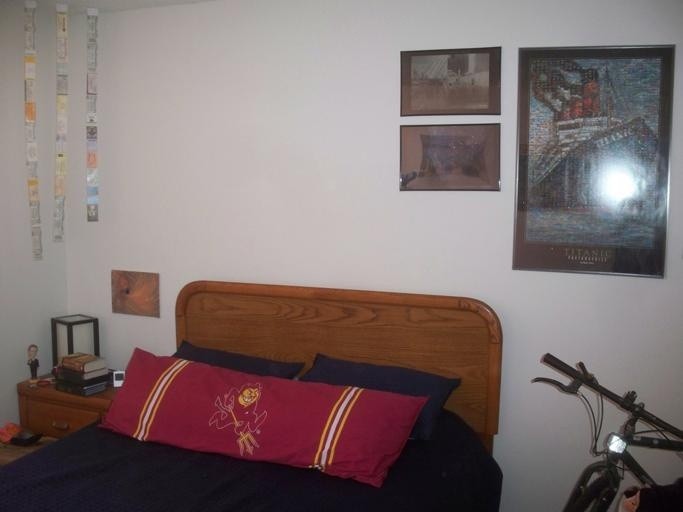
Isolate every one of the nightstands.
[18,372,120,440]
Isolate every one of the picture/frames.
[512,44,674,277]
[400,47,500,116]
[400,123,500,190]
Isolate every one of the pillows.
[296,353,462,477]
[172,342,304,378]
[100,347,428,488]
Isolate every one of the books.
[51,352,108,396]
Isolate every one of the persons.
[26,344,39,379]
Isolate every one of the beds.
[0,282,502,512]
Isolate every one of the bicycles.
[532,353,683,511]
